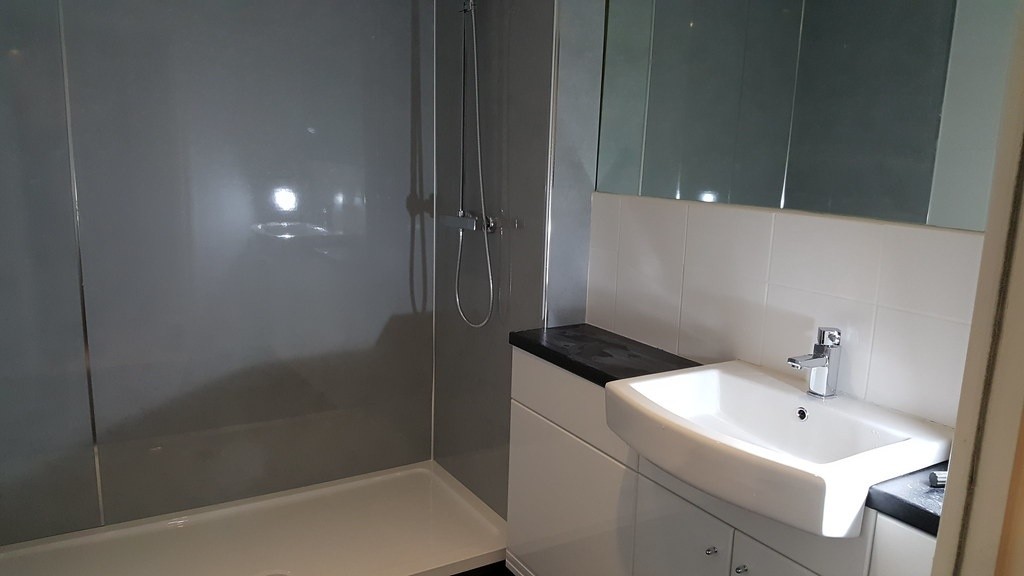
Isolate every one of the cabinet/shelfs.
[502,323,945,576]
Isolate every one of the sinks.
[605,363,955,536]
[248,219,344,242]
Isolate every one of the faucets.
[787,328,841,396]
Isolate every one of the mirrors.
[593,0,1017,230]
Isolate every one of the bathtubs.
[1,459,509,574]
